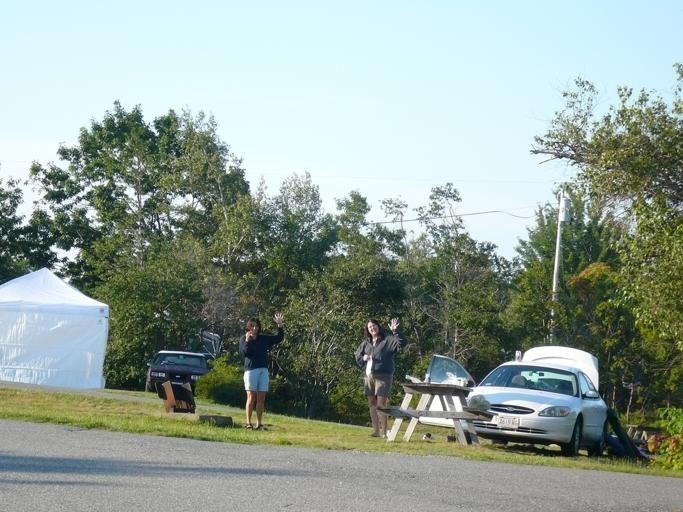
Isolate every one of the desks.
[386,383,480,445]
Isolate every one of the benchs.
[376,406,498,446]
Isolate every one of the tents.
[0,264,113,390]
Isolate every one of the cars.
[144,348,208,393]
[416,345,609,455]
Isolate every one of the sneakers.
[245,424,267,431]
[369,430,386,439]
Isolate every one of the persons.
[353,317,403,440]
[237,312,285,433]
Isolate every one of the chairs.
[555,380,573,395]
[508,375,529,389]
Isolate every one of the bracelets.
[391,329,398,333]
[277,322,282,325]
